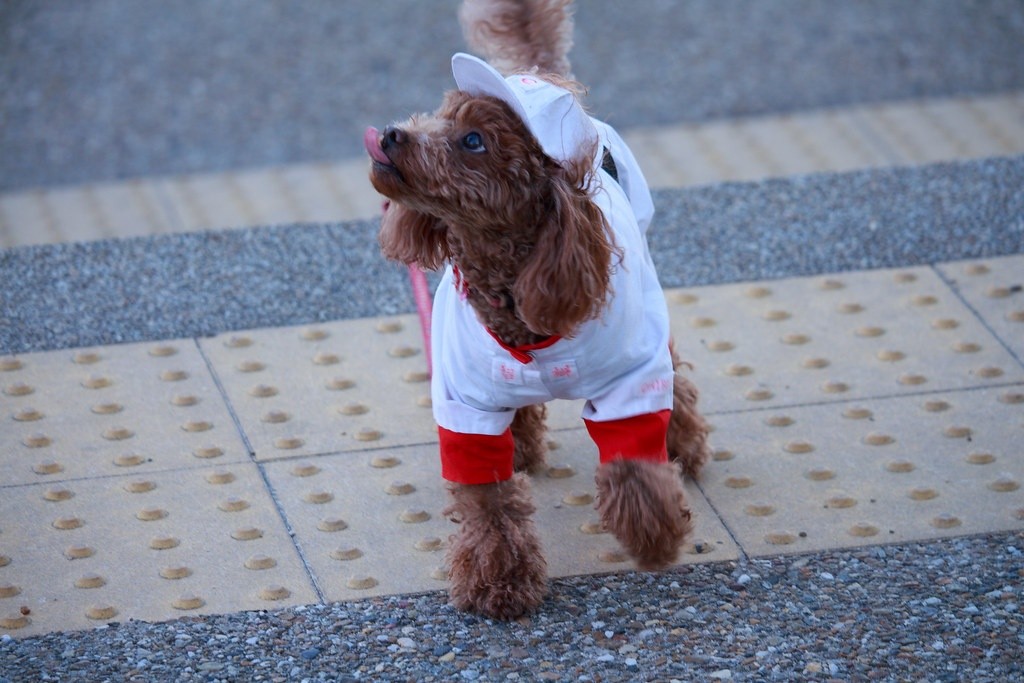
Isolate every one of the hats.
[452,52,600,184]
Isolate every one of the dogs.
[364,1,711,626]
